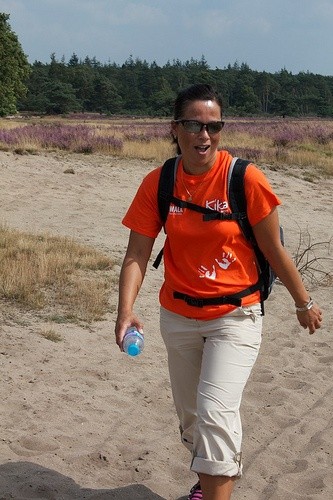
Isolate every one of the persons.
[113,83,323,500]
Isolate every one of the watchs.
[295,296,314,312]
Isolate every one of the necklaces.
[180,154,219,204]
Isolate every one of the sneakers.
[188,480,203,499]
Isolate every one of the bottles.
[123,323,144,358]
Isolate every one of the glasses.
[175,119,225,134]
